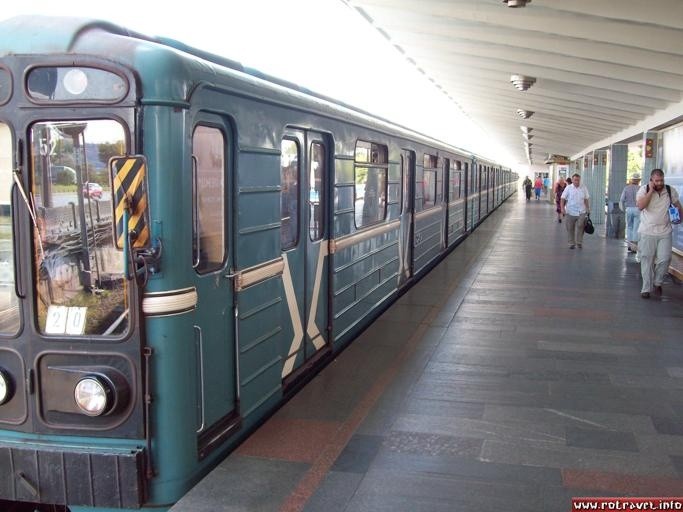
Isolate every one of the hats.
[631,173,641,180]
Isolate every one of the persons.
[560,174,591,249]
[534,176,543,201]
[522,176,533,201]
[635,169,683,298]
[554,176,567,223]
[620,172,641,253]
[565,177,572,185]
[543,176,550,194]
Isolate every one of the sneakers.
[570,244,582,248]
[653,285,662,295]
[559,219,562,223]
[642,293,648,298]
[628,246,637,253]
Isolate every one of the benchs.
[624,237,681,288]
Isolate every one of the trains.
[1,19,519,507]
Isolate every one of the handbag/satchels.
[585,215,594,234]
